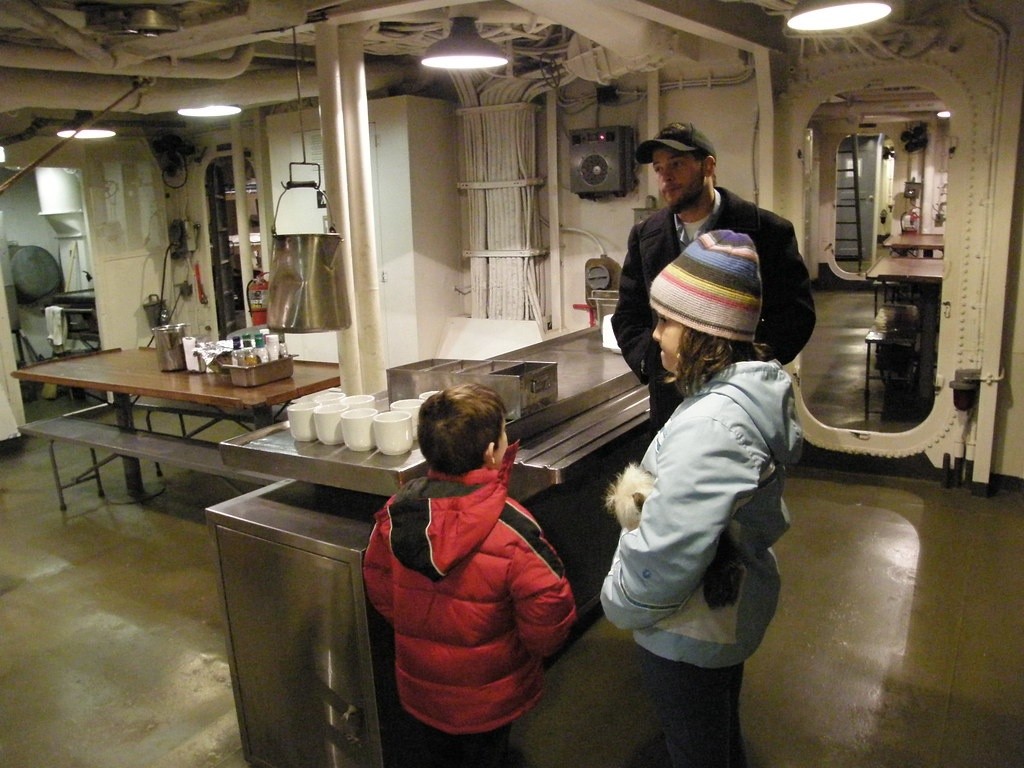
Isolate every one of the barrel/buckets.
[265,181,352,332]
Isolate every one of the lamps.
[58,108,117,139]
[421,16,508,72]
[787,0,894,32]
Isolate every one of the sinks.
[50,288,95,303]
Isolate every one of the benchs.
[17,399,305,510]
[874,280,938,316]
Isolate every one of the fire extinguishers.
[246,270,269,325]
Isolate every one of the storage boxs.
[384,357,558,416]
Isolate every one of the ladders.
[835,131,864,269]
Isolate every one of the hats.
[635,121,717,164]
[646,230,762,342]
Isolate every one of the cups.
[286,390,443,455]
[153,323,192,372]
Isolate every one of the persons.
[363,383,577,768]
[601,231,804,768]
[610,123,815,701]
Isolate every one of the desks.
[11,350,339,505]
[863,302,919,421]
[866,254,944,321]
[883,232,944,298]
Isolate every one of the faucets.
[82,271,93,282]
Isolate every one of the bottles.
[259,328,269,347]
[265,335,280,361]
[254,333,268,364]
[242,334,257,366]
[231,336,245,366]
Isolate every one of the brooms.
[41,241,77,400]
